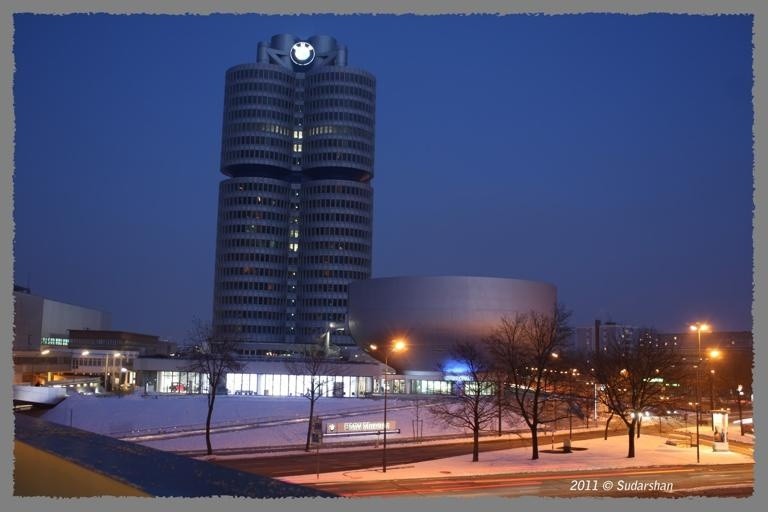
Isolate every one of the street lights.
[708,349,744,436]
[687,321,711,359]
[29,347,119,393]
[551,352,573,440]
[380,341,404,473]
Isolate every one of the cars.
[168,381,186,393]
